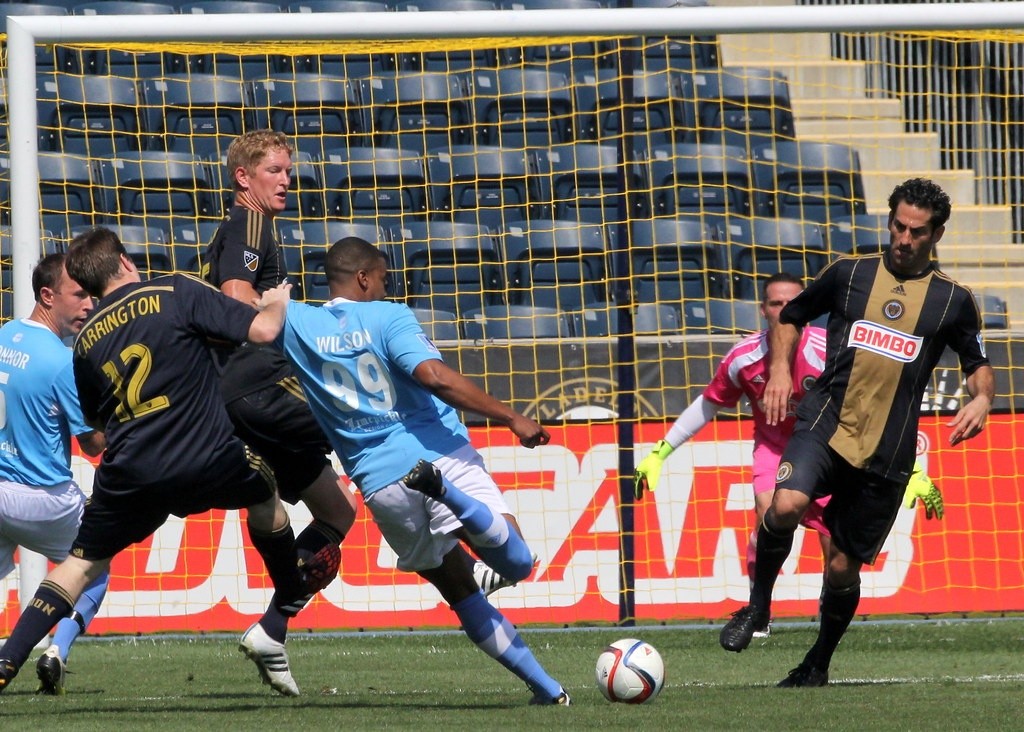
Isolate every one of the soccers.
[594,638,666,703]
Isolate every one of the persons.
[719,176,998,687]
[0,229,294,697]
[266,238,577,707]
[195,130,358,701]
[0,253,111,700]
[631,272,948,652]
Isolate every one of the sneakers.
[0,656,18,691]
[525,682,572,705]
[237,621,300,697]
[719,603,770,652]
[274,542,340,617]
[448,551,537,610]
[403,459,442,497]
[33,644,65,696]
[777,663,828,688]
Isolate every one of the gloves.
[903,461,944,521]
[632,439,673,500]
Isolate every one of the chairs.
[0,0,1011,340]
